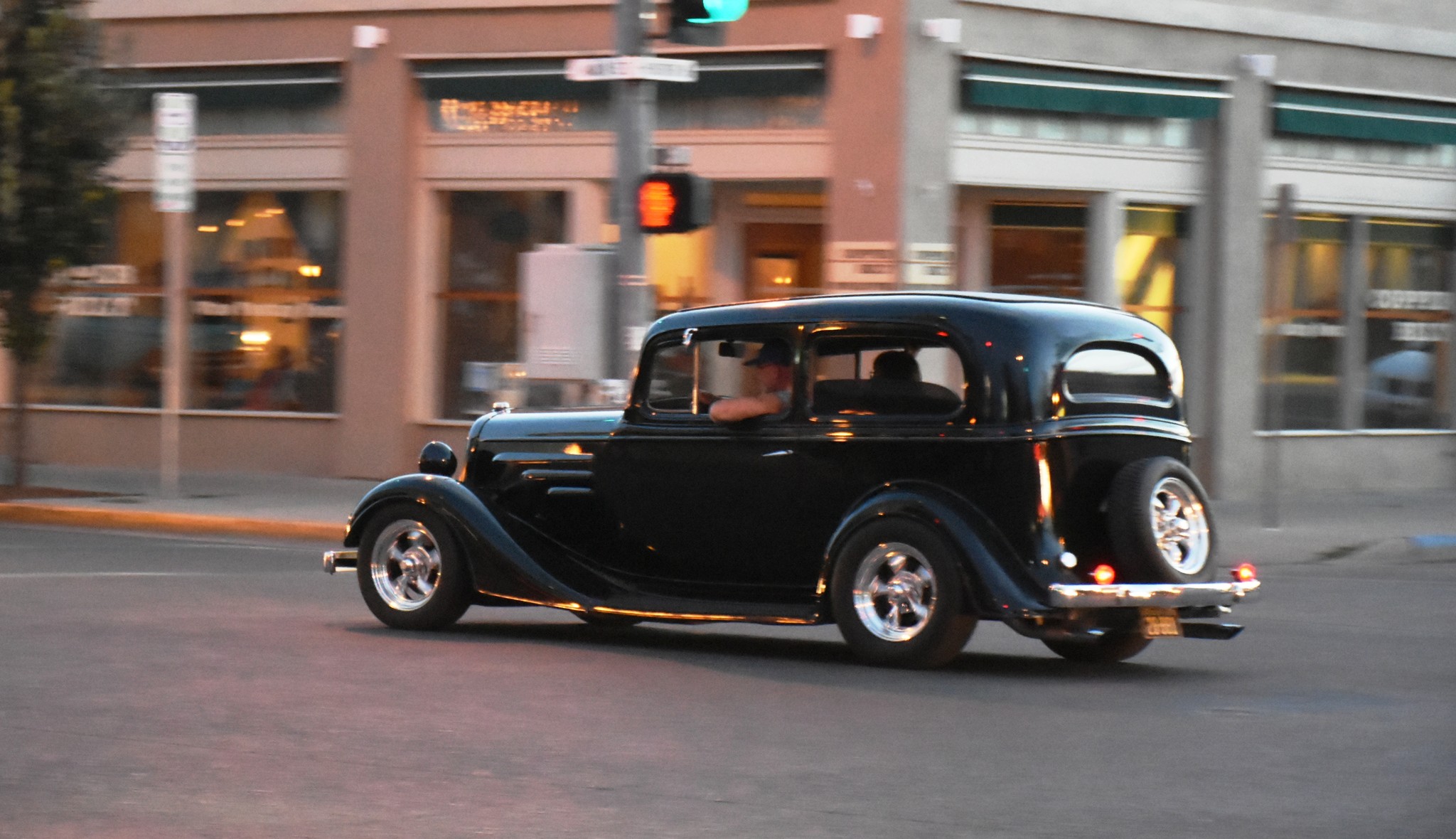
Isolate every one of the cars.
[1365,339,1436,424]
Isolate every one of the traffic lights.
[657,0,749,34]
[635,173,712,236]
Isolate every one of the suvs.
[325,290,1262,666]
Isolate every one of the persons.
[694,335,800,424]
[868,349,925,385]
